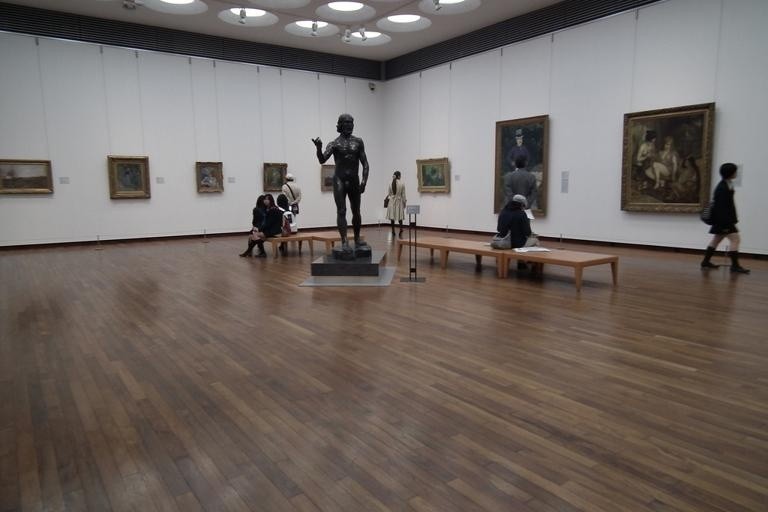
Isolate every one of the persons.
[248,195,266,249]
[491,194,544,270]
[506,129,531,169]
[700,162,752,273]
[385,170,407,235]
[282,173,301,218]
[503,152,537,211]
[636,131,700,201]
[311,115,370,250]
[277,194,297,249]
[239,194,283,258]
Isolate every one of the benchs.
[503,247,618,291]
[397,237,504,278]
[261,232,313,258]
[309,232,363,257]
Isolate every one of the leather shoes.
[239,251,256,257]
[255,252,267,258]
[701,260,719,268]
[730,266,751,273]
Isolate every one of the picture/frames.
[107,155,150,200]
[416,158,449,193]
[321,164,339,192]
[196,162,223,192]
[263,162,288,192]
[621,102,715,213]
[0,159,53,194]
[494,114,549,218]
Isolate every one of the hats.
[513,194,528,206]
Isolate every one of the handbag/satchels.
[491,230,511,250]
[282,219,290,237]
[700,203,713,224]
[384,195,389,207]
[292,203,299,213]
